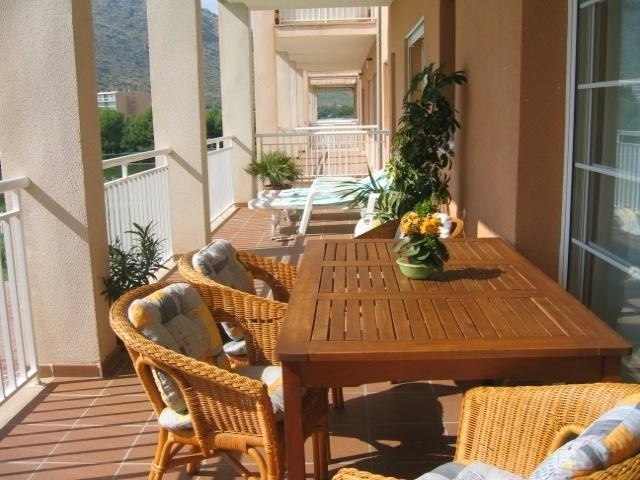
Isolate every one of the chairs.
[332,380,639,480]
[176,237,346,413]
[448,215,465,238]
[247,167,394,241]
[108,278,330,479]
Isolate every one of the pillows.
[126,282,233,411]
[432,213,450,238]
[191,238,258,298]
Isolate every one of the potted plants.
[243,150,304,189]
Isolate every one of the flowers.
[390,210,449,272]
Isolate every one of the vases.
[395,256,436,280]
